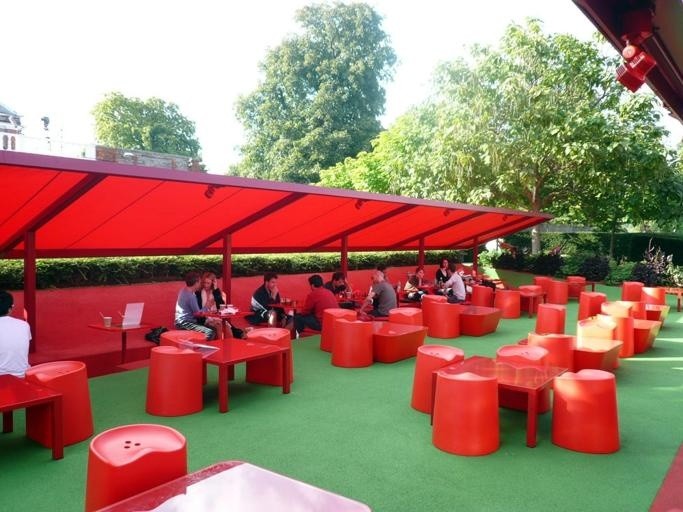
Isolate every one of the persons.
[1,291,32,381]
[439,263,465,303]
[359,270,397,318]
[434,258,451,287]
[173,272,216,341]
[244,271,294,328]
[376,264,397,293]
[195,272,233,340]
[285,275,340,339]
[403,265,429,301]
[323,272,351,299]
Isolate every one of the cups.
[102,316,112,328]
[279,297,291,304]
[346,292,352,299]
[218,304,234,314]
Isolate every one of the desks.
[0,369,64,462]
[89,298,316,369]
[175,337,292,414]
[92,460,372,512]
[432,354,569,449]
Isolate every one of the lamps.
[613,24,657,92]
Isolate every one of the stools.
[83,265,682,458]
[25,359,94,448]
[245,327,293,387]
[495,344,550,415]
[81,425,189,512]
[316,265,682,368]
[550,367,621,454]
[159,330,206,387]
[431,368,502,457]
[144,345,204,417]
[410,343,465,416]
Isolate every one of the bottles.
[396,281,401,292]
[438,277,443,288]
